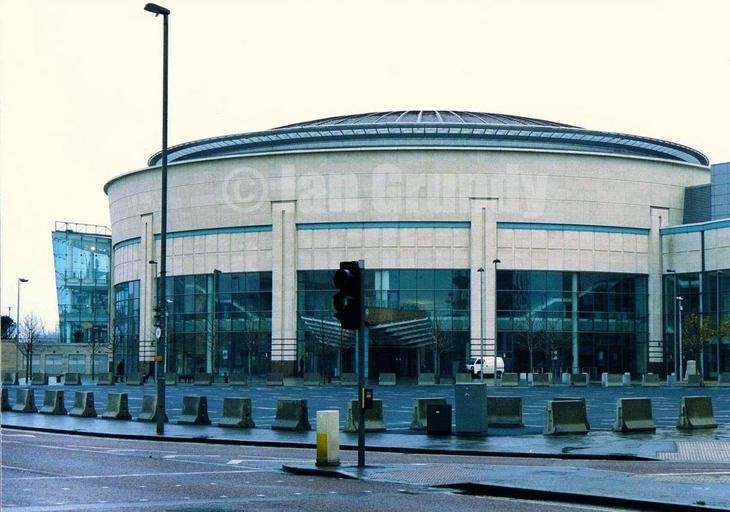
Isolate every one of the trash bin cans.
[428,404,452,433]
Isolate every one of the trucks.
[466,356,504,379]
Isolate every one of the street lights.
[477,267,484,382]
[144,3,170,435]
[148,260,160,327]
[676,296,684,382]
[493,259,501,379]
[14,278,28,385]
[666,269,677,380]
[90,246,96,382]
[717,271,727,383]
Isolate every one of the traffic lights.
[333,261,362,330]
[153,307,162,318]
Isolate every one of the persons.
[115,359,125,382]
[300,355,304,377]
